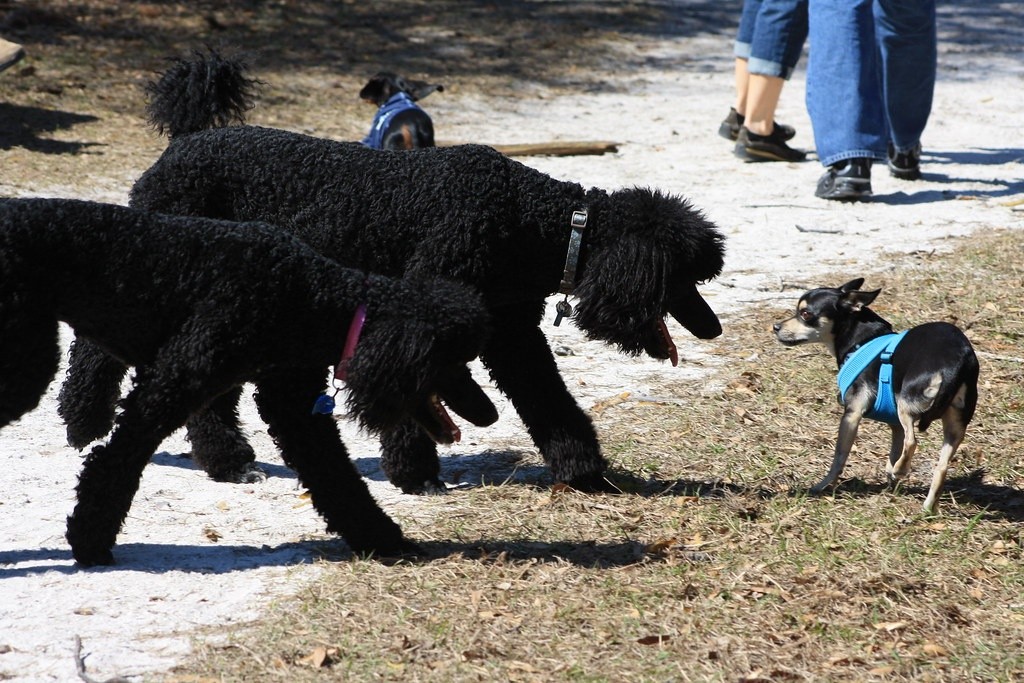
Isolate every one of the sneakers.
[718,106,796,142]
[815,157,874,199]
[886,136,923,180]
[734,124,807,162]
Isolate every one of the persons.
[806,0,939,202]
[717,0,807,162]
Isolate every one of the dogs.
[59,58,726,491]
[359,71,445,148]
[0,195,499,566]
[774,278,980,514]
